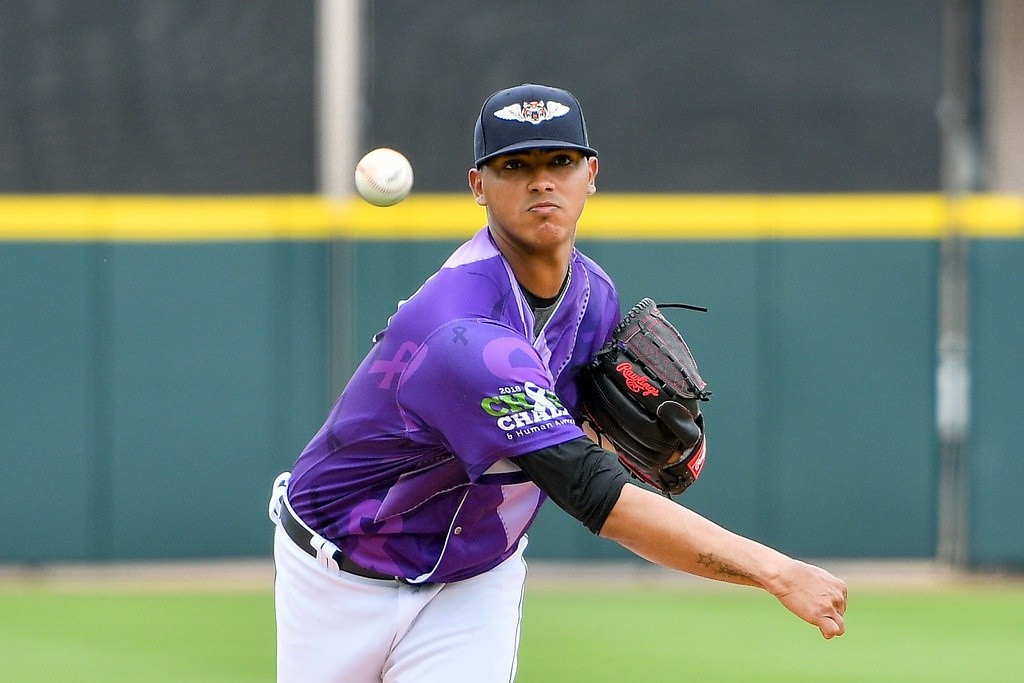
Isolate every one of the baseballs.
[354,148,417,207]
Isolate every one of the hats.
[474,83,598,172]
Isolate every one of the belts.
[280,499,434,587]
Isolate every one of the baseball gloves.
[581,296,710,494]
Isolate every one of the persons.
[270,83,848,683]
